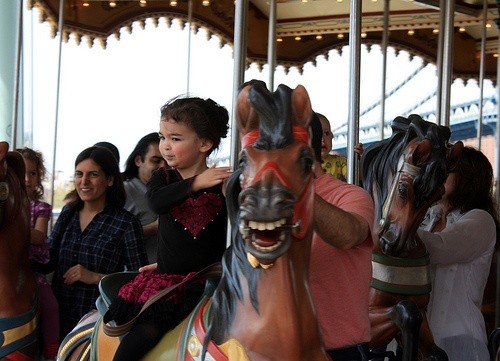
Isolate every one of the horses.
[57,79,330,361]
[357,113,464,361]
[0,141,43,361]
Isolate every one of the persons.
[315,111,363,183]
[31,146,148,344]
[113,96,233,361]
[417,146,497,361]
[121,132,173,264]
[14,147,59,361]
[306,110,380,361]
